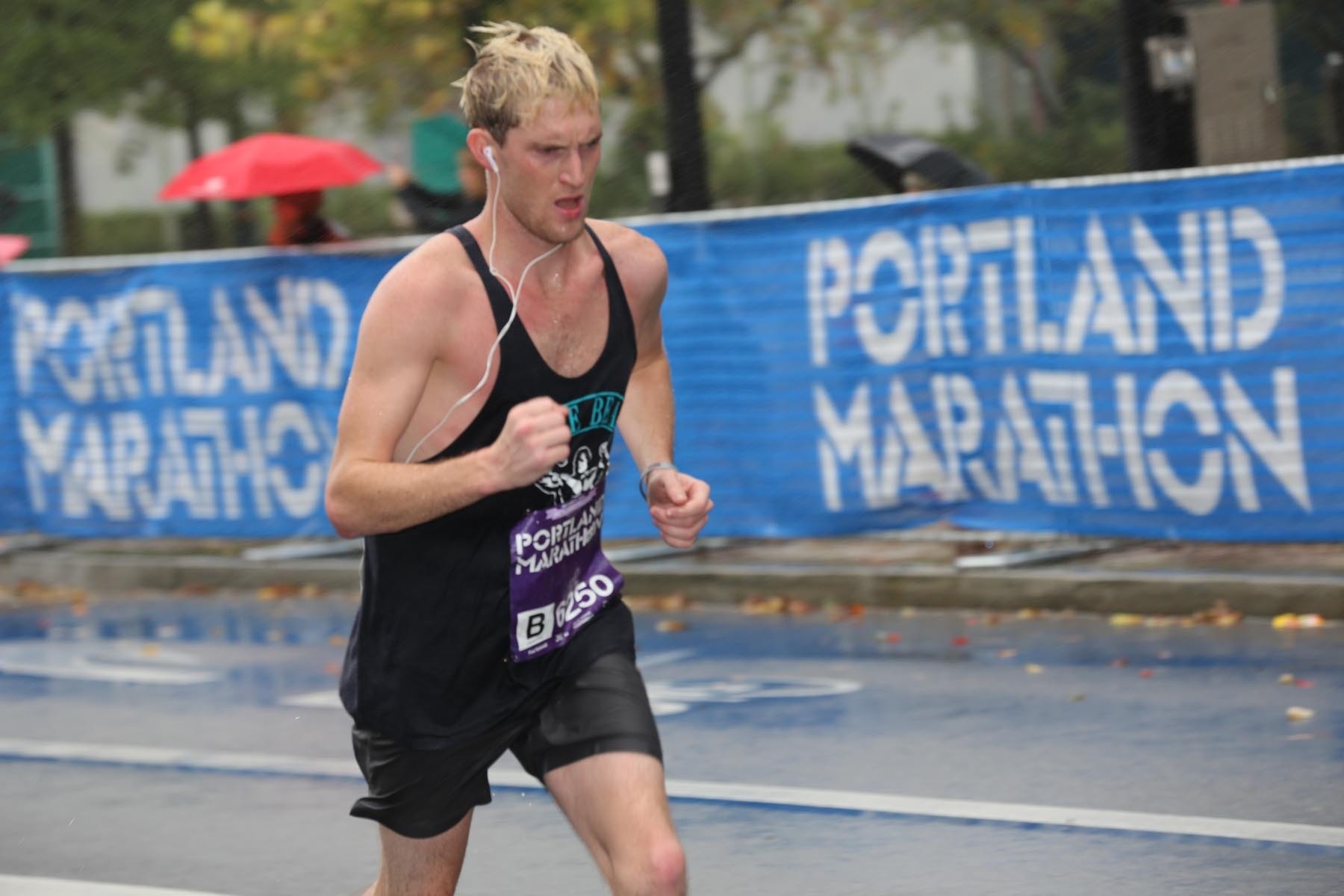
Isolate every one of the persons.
[395,176,460,235]
[156,136,383,201]
[321,20,714,896]
[269,190,342,244]
[893,172,941,191]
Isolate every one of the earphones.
[482,146,500,173]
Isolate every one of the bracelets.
[636,463,671,497]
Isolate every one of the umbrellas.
[0,234,32,263]
[846,137,990,191]
[408,115,474,193]
